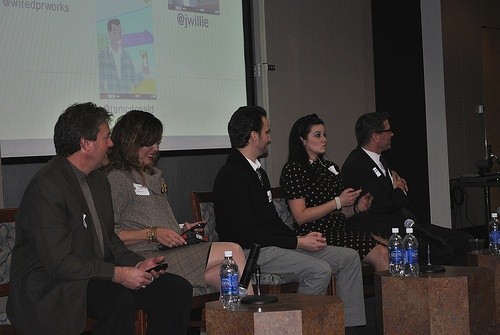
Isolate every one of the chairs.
[189,296,218,331]
[0,208,148,335]
[191,191,300,295]
[271,187,295,230]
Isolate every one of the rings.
[142,285,146,289]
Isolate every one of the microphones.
[238,243,261,299]
[404,219,445,248]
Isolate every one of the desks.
[201,294,345,335]
[374,265,495,335]
[459,178,500,233]
[477,250,500,335]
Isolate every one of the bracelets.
[353,204,359,213]
[146,227,158,243]
[334,197,341,210]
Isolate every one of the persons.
[212,105,367,327]
[107,111,254,297]
[280,112,476,270]
[7,101,192,335]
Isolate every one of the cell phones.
[181,223,207,237]
[146,263,168,273]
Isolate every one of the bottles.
[403,228,419,276]
[488,211,500,255]
[220,250,240,310]
[387,228,405,277]
